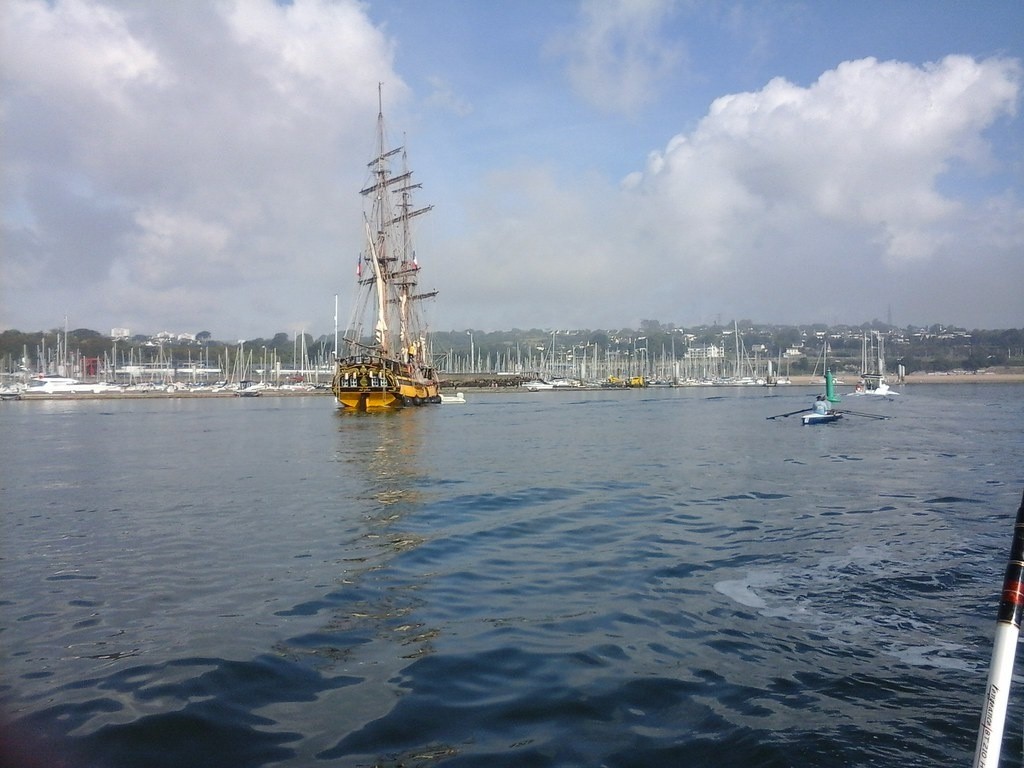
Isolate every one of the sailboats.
[856,328,886,398]
[332,83,441,412]
[0,315,793,400]
[896,364,906,386]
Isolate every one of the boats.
[801,410,843,425]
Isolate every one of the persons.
[408,343,415,363]
[813,395,831,414]
[856,381,872,392]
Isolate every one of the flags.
[413,251,417,270]
[357,256,362,277]
